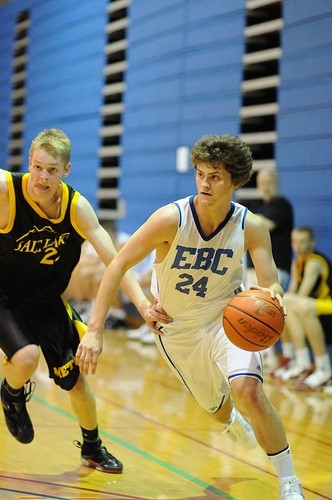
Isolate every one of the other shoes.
[127,324,156,344]
[262,348,331,393]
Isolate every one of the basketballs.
[222,290,285,352]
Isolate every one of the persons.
[74,135,306,500]
[61,169,332,394]
[0,130,173,473]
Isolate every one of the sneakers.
[1,378,36,443]
[73,439,122,474]
[222,409,256,449]
[280,475,305,500]
